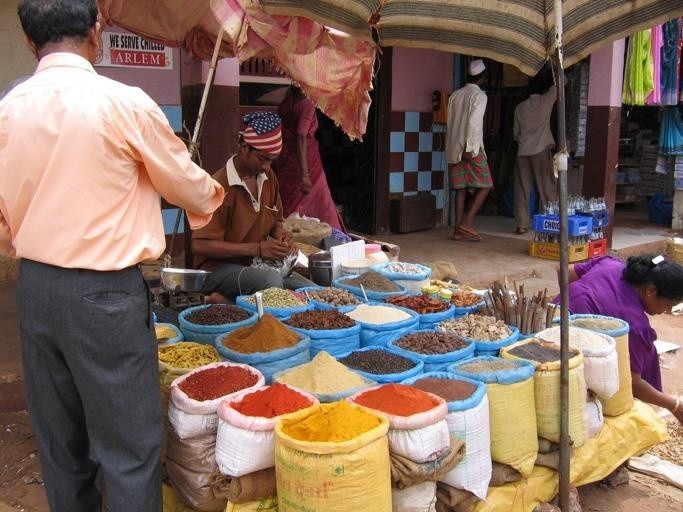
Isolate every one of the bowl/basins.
[160,265,212,293]
[306,249,332,286]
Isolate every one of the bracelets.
[671,399,680,414]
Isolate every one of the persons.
[270,84,348,235]
[445,59,494,241]
[0,0,225,512]
[513,74,569,234]
[191,112,318,297]
[550,253,683,428]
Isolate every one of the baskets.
[528,210,609,263]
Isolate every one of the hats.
[467,59,486,76]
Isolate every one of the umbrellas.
[256,0,683,512]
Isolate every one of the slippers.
[455,225,483,242]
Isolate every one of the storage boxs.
[648,194,674,224]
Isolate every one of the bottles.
[531,195,609,259]
